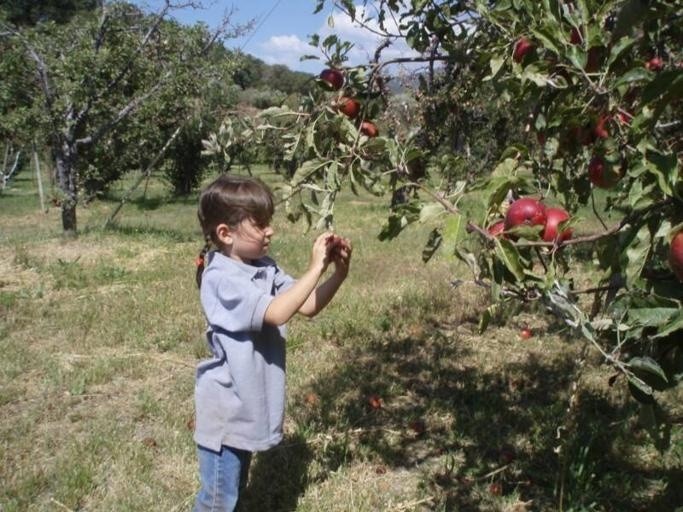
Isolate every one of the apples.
[361,122,379,138]
[670,231,683,277]
[407,418,425,437]
[527,44,683,189]
[414,446,539,502]
[541,210,573,251]
[569,25,587,43]
[512,38,538,58]
[507,377,524,393]
[337,97,361,116]
[486,221,506,242]
[557,385,647,441]
[369,395,385,415]
[520,328,534,338]
[505,198,546,240]
[377,467,388,474]
[319,70,343,91]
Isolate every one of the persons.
[188,172,352,512]
